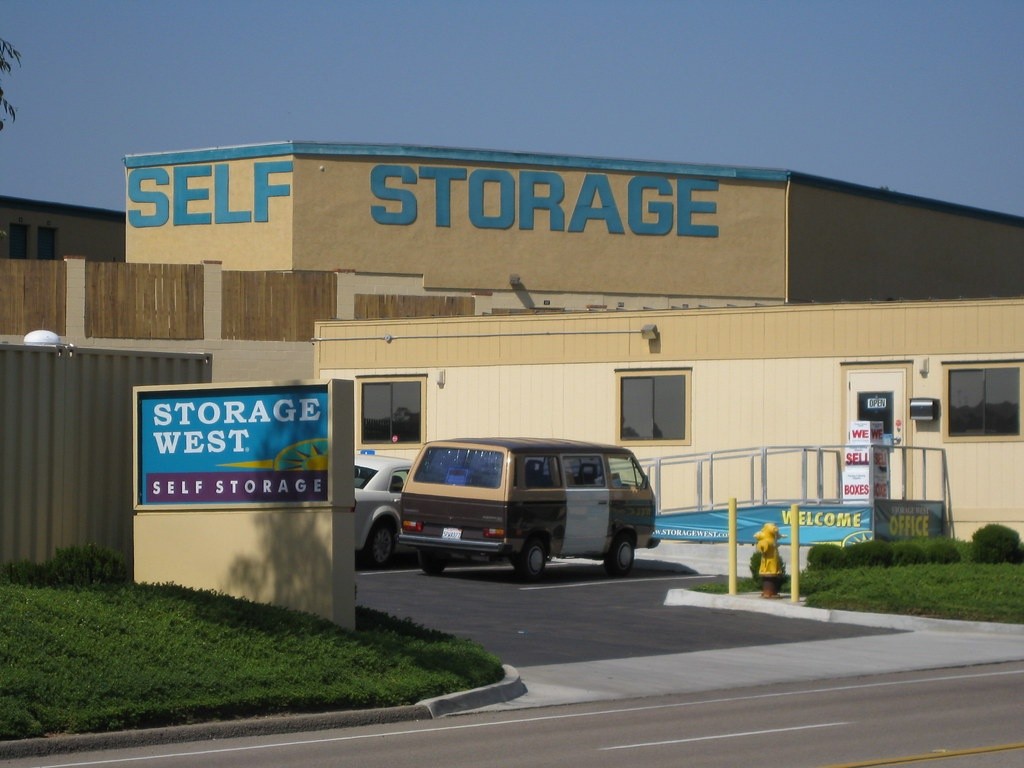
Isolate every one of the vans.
[395,438,664,584]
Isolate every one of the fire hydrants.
[753,522,785,599]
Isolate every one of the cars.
[352,453,428,570]
